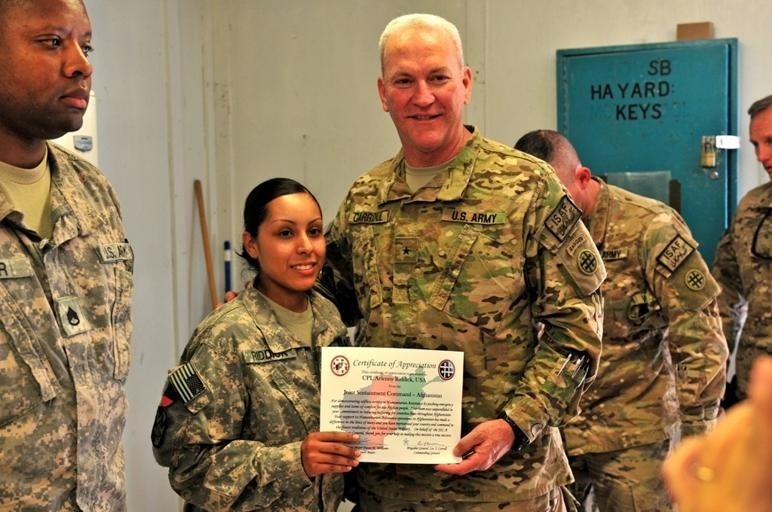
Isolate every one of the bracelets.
[499,410,529,453]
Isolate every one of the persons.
[0,1,136,512]
[707,94,772,405]
[659,353,771,512]
[513,128,731,512]
[216,12,607,512]
[151,175,361,512]
